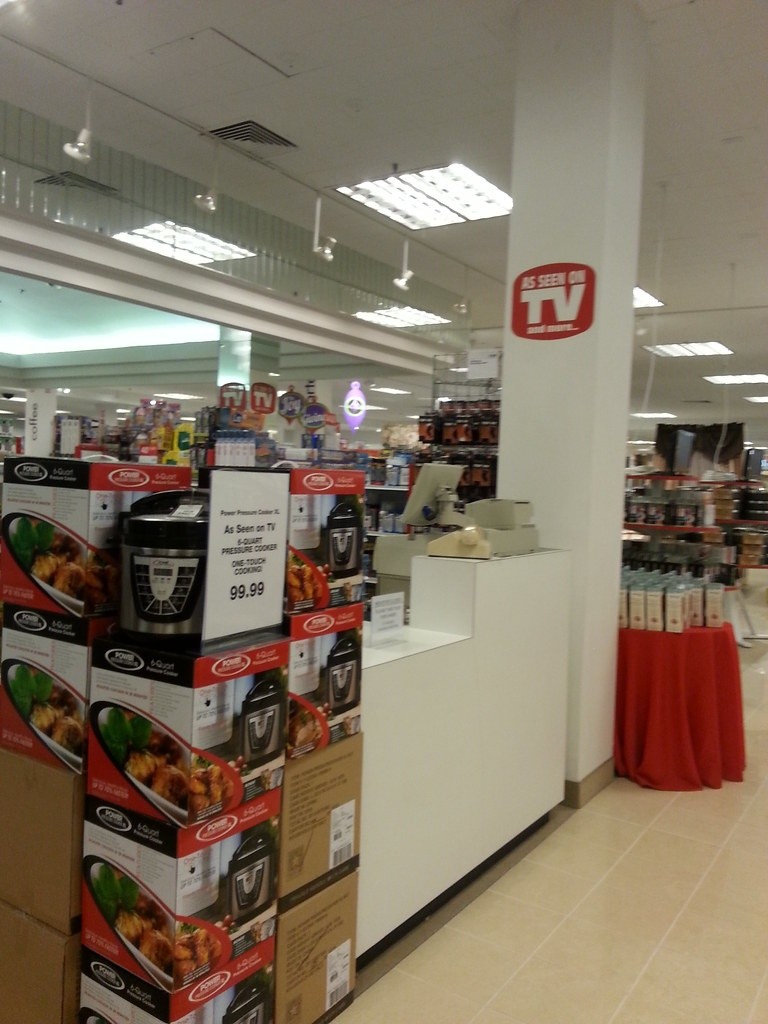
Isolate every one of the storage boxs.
[0,399,768,1024]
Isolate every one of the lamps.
[312,192,337,262]
[60,78,96,164]
[392,233,414,291]
[453,298,469,314]
[193,141,220,215]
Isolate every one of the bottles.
[215,430,255,467]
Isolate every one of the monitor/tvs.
[400,463,465,526]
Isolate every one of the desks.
[615,621,748,790]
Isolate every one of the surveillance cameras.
[2,393,14,399]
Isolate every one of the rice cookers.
[227,831,276,926]
[240,679,284,770]
[118,489,211,648]
[325,636,360,715]
[222,985,264,1024]
[327,502,360,578]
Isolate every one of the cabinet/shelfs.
[365,462,422,586]
[624,472,723,532]
[0,411,16,466]
[698,481,768,586]
